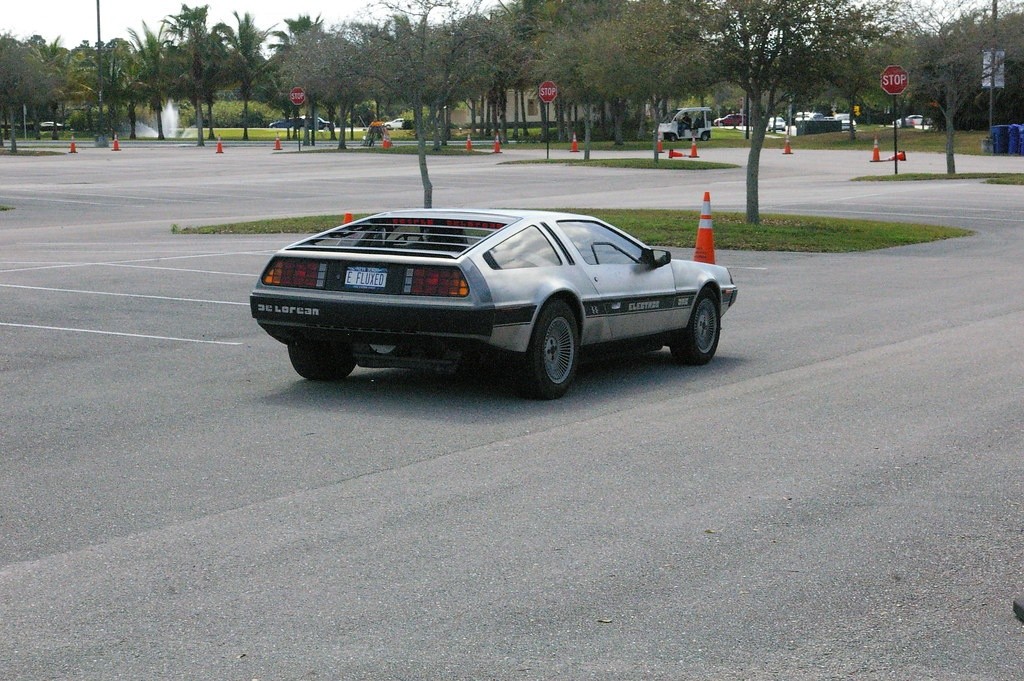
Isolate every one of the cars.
[714,111,856,133]
[384,118,406,130]
[248,206,739,401]
[893,114,933,126]
[41,122,70,131]
[269,117,336,131]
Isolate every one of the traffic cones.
[781,137,793,155]
[465,133,473,152]
[687,135,700,159]
[383,132,393,148]
[887,150,907,161]
[491,133,504,154]
[110,132,121,152]
[669,148,687,159]
[570,131,581,153]
[869,134,886,163]
[68,134,78,153]
[657,132,665,154]
[214,134,224,154]
[691,190,716,267]
[273,133,283,150]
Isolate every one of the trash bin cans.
[990,125,1009,153]
[1009,124,1024,155]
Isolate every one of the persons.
[678,112,691,136]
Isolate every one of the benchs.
[682,117,694,137]
[695,127,704,136]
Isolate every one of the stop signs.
[880,64,910,96]
[290,86,306,106]
[539,81,558,103]
